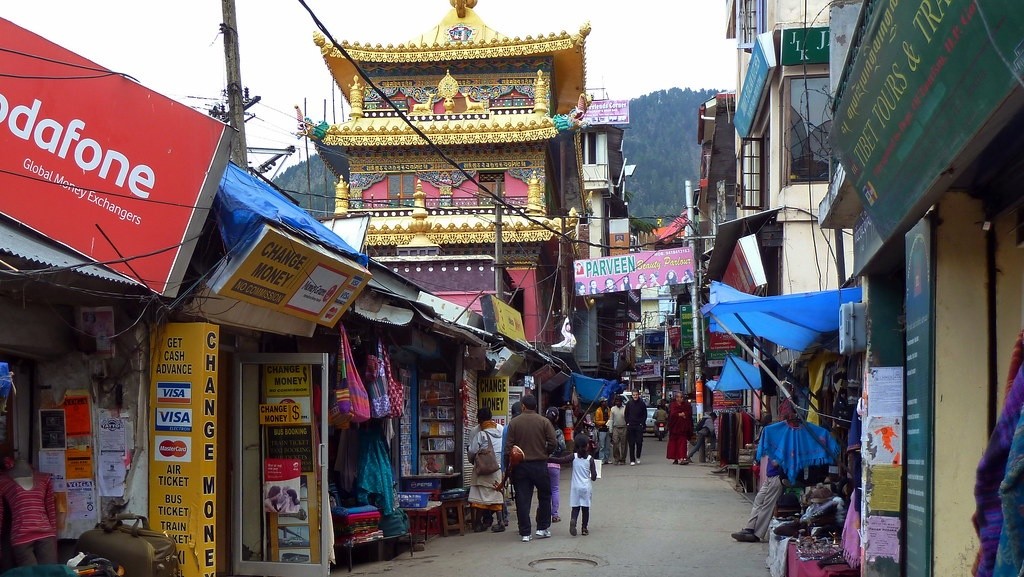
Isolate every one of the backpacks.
[696,417,711,432]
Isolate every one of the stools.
[441,497,468,537]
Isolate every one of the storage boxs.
[396,491,431,508]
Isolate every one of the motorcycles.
[653,416,669,441]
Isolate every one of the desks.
[771,518,861,577]
[404,501,442,541]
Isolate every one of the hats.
[481,421,497,430]
[599,396,608,401]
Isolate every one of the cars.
[644,407,661,433]
[617,391,633,405]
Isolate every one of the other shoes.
[731,527,760,542]
[570,519,577,536]
[600,458,640,466]
[474,516,508,532]
[552,516,561,522]
[673,459,689,465]
[582,527,589,535]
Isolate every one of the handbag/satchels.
[777,486,800,508]
[474,432,499,475]
[75,514,179,577]
[834,379,853,428]
[330,321,403,429]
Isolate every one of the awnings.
[564,372,626,403]
[705,351,771,422]
[700,280,863,351]
[0,285,572,383]
[700,206,783,280]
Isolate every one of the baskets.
[441,489,467,500]
[396,491,433,508]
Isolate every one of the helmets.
[657,404,661,409]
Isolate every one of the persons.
[466,394,597,542]
[664,269,693,285]
[575,262,618,295]
[666,393,693,467]
[621,273,660,291]
[595,389,668,466]
[731,457,785,543]
[687,412,717,463]
[0,456,57,567]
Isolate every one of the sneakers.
[536,528,551,537]
[522,534,532,541]
